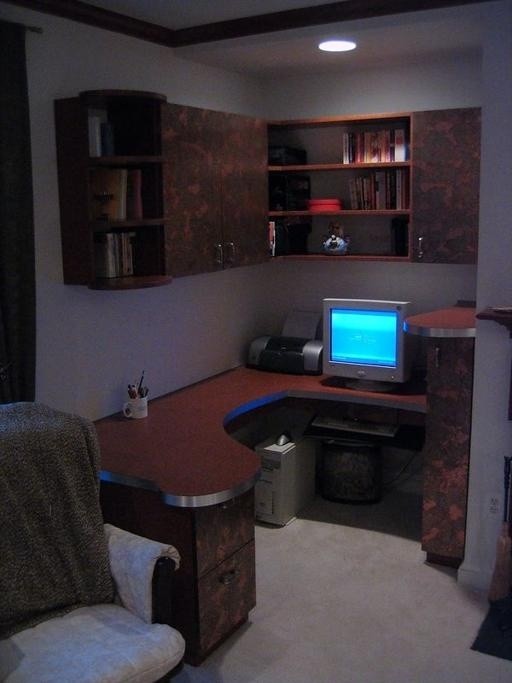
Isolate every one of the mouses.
[277,430,291,446]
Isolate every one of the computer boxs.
[254,435,316,526]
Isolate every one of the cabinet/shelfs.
[266,113,408,263]
[158,98,268,280]
[53,88,172,292]
[408,107,478,264]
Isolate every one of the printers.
[247,309,323,375]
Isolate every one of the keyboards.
[312,415,399,438]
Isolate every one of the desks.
[76,360,427,668]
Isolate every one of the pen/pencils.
[128,370,149,399]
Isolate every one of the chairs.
[0,396,190,683]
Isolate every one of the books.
[87,166,146,222]
[94,231,141,278]
[86,110,118,157]
[341,125,410,212]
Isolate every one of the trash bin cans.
[316,439,383,505]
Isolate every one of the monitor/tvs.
[322,298,410,392]
